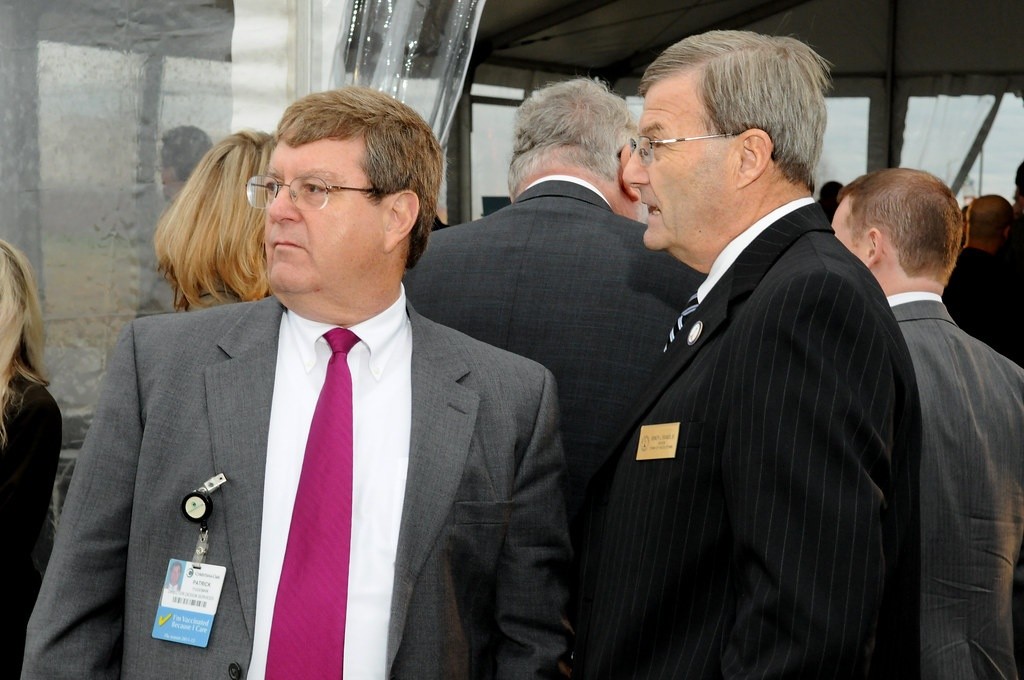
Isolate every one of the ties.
[665,292,701,353]
[263,327,361,680]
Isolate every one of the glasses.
[629,131,776,167]
[246,175,382,211]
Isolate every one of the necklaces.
[184,290,240,310]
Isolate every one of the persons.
[0,237,63,680]
[160,123,214,202]
[21,87,574,680]
[164,561,182,593]
[810,163,1024,368]
[403,76,706,494]
[566,28,922,680]
[830,167,1024,680]
[150,131,277,313]
